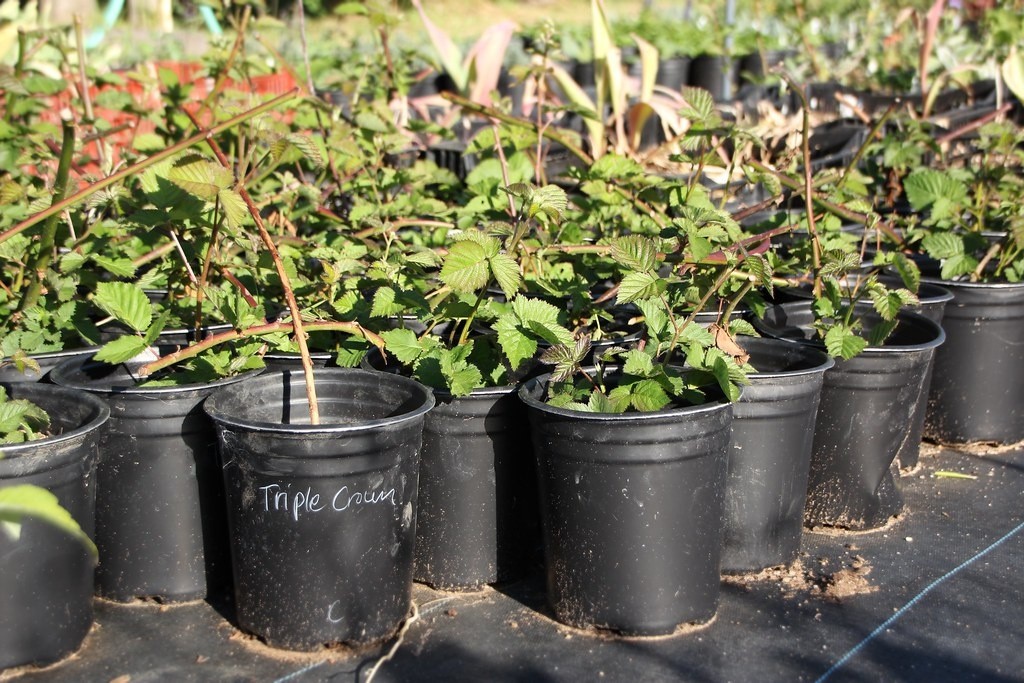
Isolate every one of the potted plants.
[0,0,1024,678]
